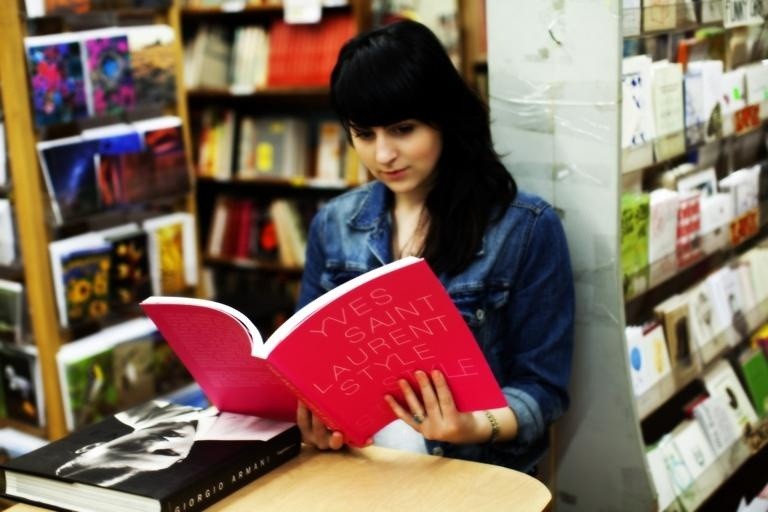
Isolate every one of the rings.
[413,410,425,423]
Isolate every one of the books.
[139,255,509,448]
[0,1,462,461]
[621,1,768,512]
[1,399,304,512]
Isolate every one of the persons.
[297,19,575,475]
[54,399,296,487]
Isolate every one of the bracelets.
[483,410,499,444]
